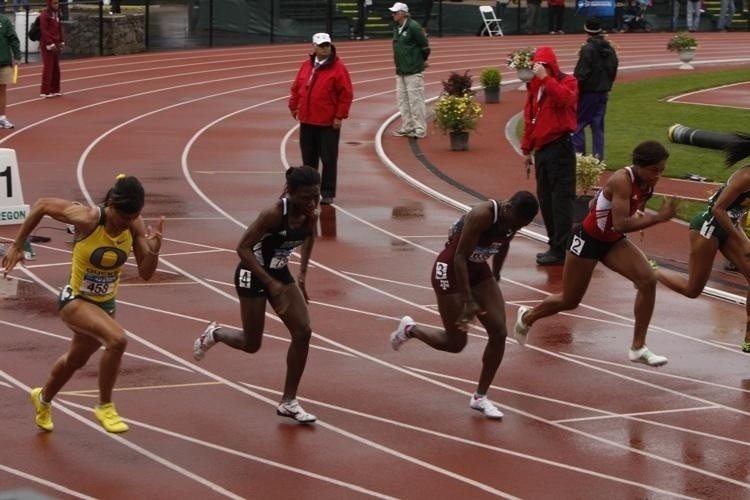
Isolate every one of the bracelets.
[149,249,162,256]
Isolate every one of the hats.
[312,32,332,46]
[583,16,602,34]
[388,2,409,13]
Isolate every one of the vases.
[516,67,536,91]
[449,131,469,151]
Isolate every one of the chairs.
[478,5,504,38]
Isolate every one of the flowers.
[432,92,483,136]
[504,46,537,71]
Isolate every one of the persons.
[387,3,433,138]
[1,173,165,436]
[288,32,355,204]
[520,44,579,264]
[717,1,749,33]
[526,0,544,35]
[633,163,749,352]
[192,163,323,424]
[512,141,681,367]
[636,0,653,11]
[38,0,66,99]
[547,1,566,35]
[667,1,702,32]
[348,0,434,39]
[0,4,21,130]
[571,16,620,162]
[391,189,540,420]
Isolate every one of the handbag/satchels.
[27,17,42,41]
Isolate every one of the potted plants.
[666,29,698,70]
[480,67,502,103]
[571,151,607,223]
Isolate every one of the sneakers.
[468,393,504,421]
[192,320,222,363]
[276,398,317,424]
[633,258,658,290]
[742,340,750,353]
[512,305,532,346]
[94,401,130,434]
[39,92,62,98]
[391,128,408,136]
[30,387,55,433]
[407,131,427,138]
[390,315,414,351]
[0,114,14,130]
[319,196,333,204]
[629,344,668,367]
